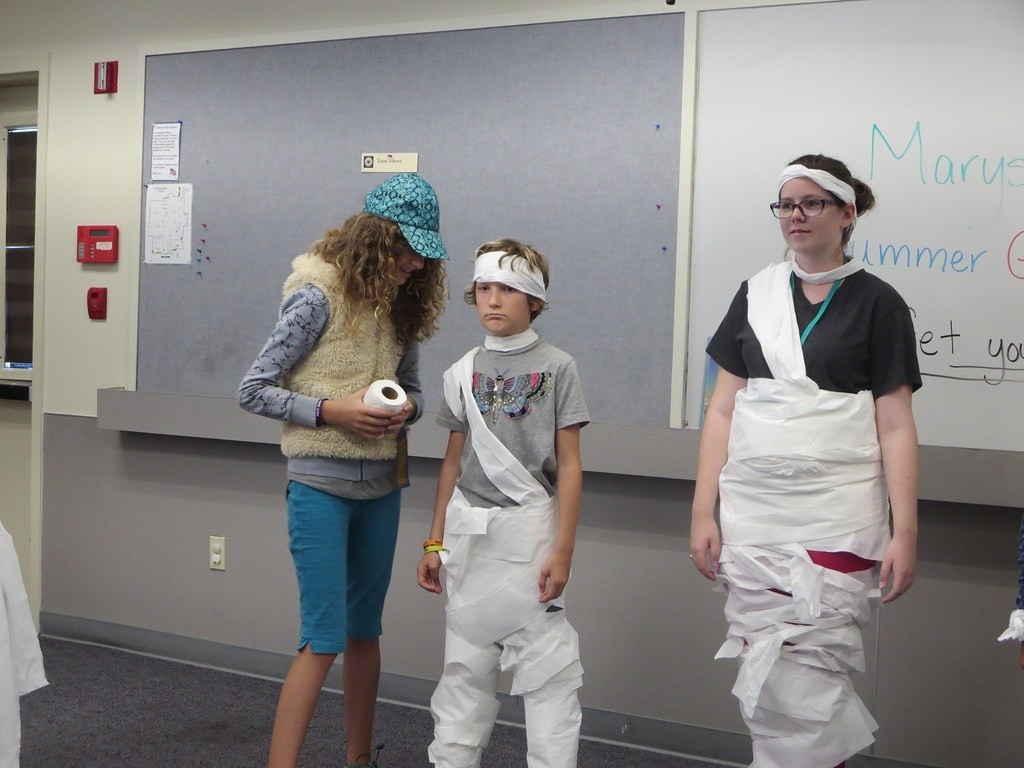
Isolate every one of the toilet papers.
[363,378,408,439]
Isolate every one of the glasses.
[770,199,845,219]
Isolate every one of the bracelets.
[424,546,443,552]
[316,398,330,426]
[423,540,443,548]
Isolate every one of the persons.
[415,238,585,768]
[238,175,451,768]
[688,154,923,768]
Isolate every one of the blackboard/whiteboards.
[684,1,1024,450]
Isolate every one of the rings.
[689,554,694,559]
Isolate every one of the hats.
[363,173,450,261]
